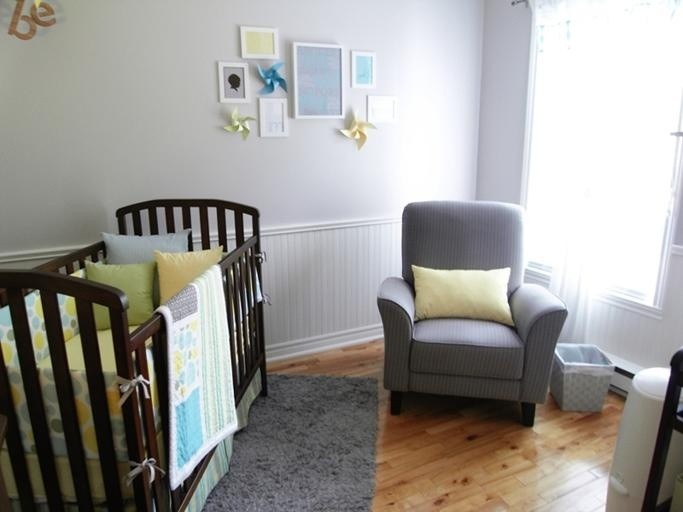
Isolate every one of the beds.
[0,198,270,512]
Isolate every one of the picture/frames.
[368,96,399,124]
[259,96,287,140]
[351,51,376,89]
[217,62,250,104]
[240,27,279,60]
[292,42,344,118]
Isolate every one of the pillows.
[411,263,512,323]
[84,260,156,327]
[100,229,190,264]
[155,247,223,305]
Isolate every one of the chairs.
[378,201,568,425]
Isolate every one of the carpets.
[205,373,380,512]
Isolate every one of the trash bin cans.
[551,344,616,412]
